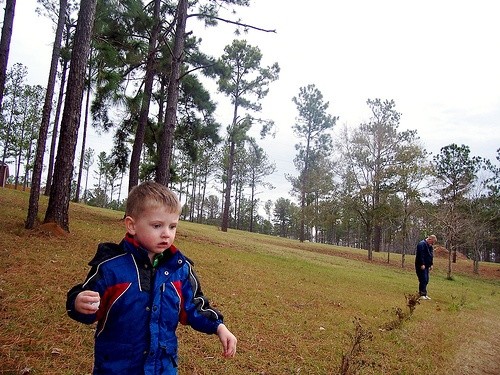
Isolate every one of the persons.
[64,182,237,374]
[415,235,437,300]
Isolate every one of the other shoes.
[418,295,431,300]
